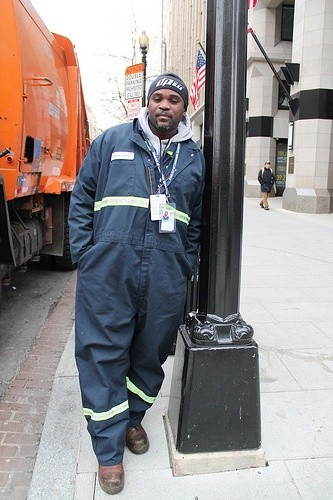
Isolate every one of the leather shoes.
[126,424,149,454]
[99,461,124,495]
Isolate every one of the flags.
[190,50,206,110]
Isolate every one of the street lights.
[138,31,150,106]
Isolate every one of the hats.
[265,161,270,164]
[147,73,188,112]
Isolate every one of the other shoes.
[264,208,269,210]
[259,202,264,208]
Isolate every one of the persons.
[163,211,169,220]
[68,74,206,494]
[258,161,274,210]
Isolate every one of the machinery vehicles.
[0,0,93,292]
